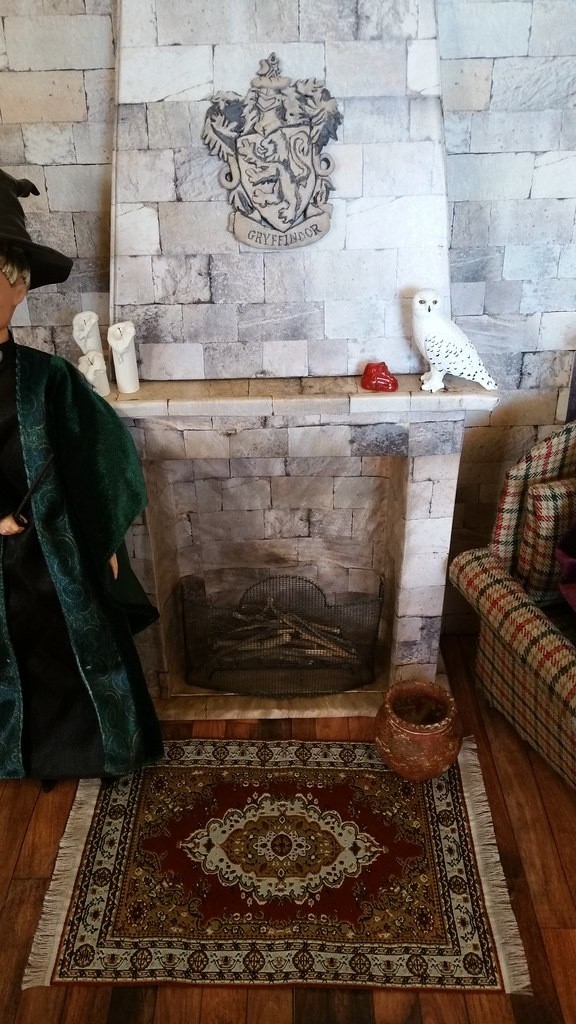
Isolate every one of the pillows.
[516,479,576,604]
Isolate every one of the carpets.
[20,732,528,996]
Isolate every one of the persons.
[0,170,161,794]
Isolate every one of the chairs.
[449,420,576,791]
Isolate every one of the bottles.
[374,679,463,783]
